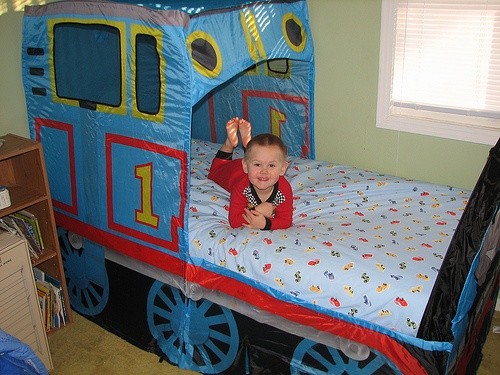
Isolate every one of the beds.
[55,137,500,375]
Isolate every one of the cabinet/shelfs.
[0,133,73,338]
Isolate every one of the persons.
[207,117,293,230]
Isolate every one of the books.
[33,268,69,332]
[0,211,44,259]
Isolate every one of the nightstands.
[0,231,54,372]
